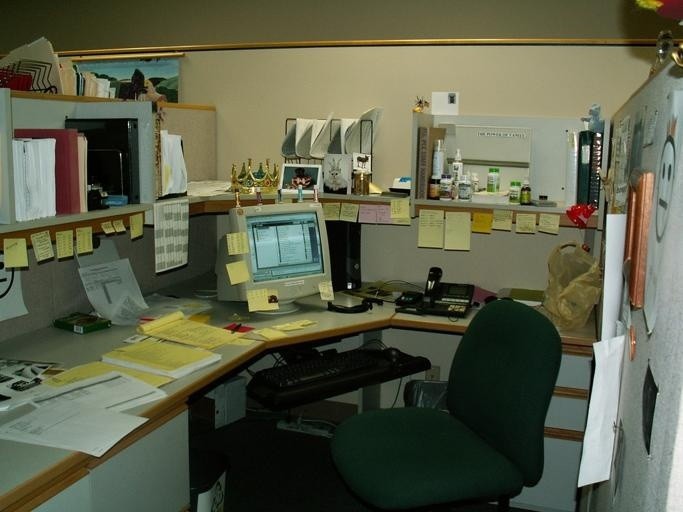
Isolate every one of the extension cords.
[276,420,331,439]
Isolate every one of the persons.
[289,167,313,190]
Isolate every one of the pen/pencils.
[231,323,241,334]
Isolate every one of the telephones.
[416,267,474,319]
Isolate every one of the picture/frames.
[624,172,654,308]
[279,163,322,193]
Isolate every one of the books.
[417,126,446,201]
[565,130,602,210]
[100,311,246,382]
[14,129,88,214]
[508,288,544,307]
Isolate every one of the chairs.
[329,301,562,512]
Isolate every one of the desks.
[0,272,607,512]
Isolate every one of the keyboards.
[256,350,378,389]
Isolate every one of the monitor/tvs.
[216,202,333,317]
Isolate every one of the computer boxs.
[326,220,362,291]
[190,432,230,512]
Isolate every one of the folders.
[13,128,81,214]
[65,118,140,204]
[579,132,594,209]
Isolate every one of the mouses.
[384,348,402,364]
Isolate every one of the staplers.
[87,190,110,211]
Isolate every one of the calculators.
[395,290,424,306]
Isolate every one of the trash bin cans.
[188,447,230,512]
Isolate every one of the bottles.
[474,177,479,191]
[313,185,319,202]
[458,175,472,201]
[297,185,304,202]
[452,149,463,181]
[429,175,441,200]
[433,138,444,179]
[275,188,283,203]
[235,188,241,207]
[440,174,453,201]
[487,168,500,191]
[520,174,532,205]
[354,170,370,195]
[255,187,264,205]
[509,181,521,204]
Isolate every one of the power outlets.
[425,366,440,381]
